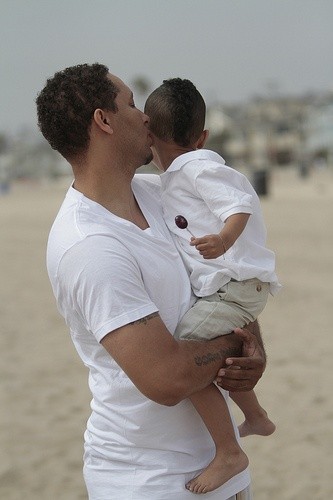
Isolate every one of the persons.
[35,64,266,500]
[144,76,277,493]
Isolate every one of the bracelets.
[218,233,228,254]
